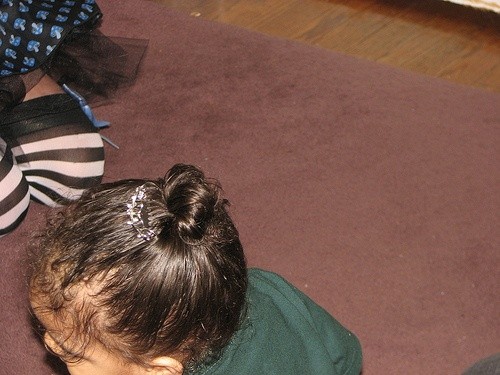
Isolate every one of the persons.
[1,1,114,234]
[29,159,365,375]
